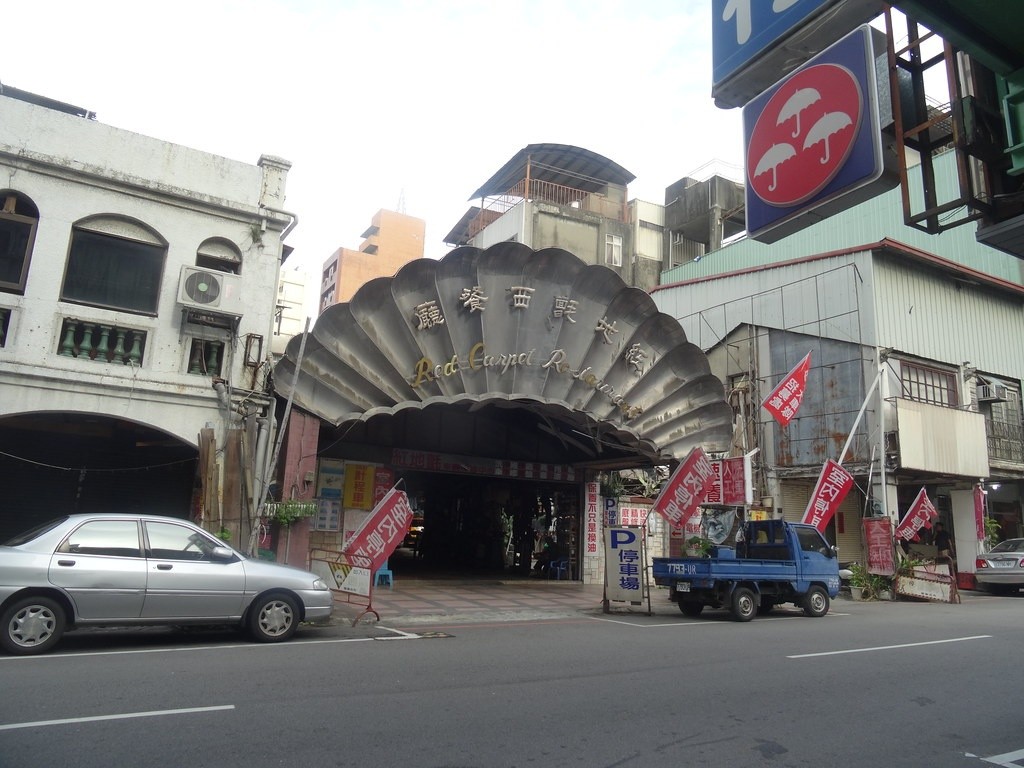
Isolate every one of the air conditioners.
[176,264,247,317]
[976,383,1007,399]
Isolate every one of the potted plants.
[249,497,318,527]
[847,562,894,600]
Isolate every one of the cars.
[0,513,334,656]
[975,537,1023,597]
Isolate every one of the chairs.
[374,558,393,587]
[533,555,569,580]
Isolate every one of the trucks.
[652,519,842,622]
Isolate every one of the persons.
[530,537,560,578]
[935,522,954,559]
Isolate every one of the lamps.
[988,481,1002,490]
